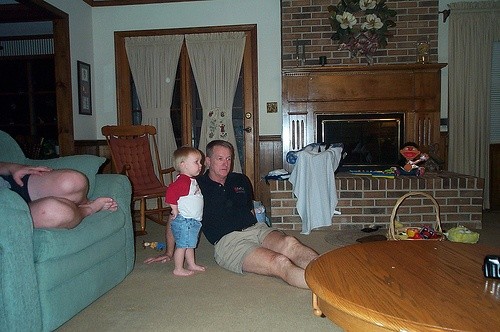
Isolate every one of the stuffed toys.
[395,142,425,177]
[446,225,479,244]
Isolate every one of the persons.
[164,146,206,276]
[143,139,321,291]
[0,161,118,229]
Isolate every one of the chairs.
[100,125,175,231]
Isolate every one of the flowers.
[328,0,396,56]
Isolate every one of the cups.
[320,56,326,65]
[417,41,429,64]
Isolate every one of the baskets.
[387,191,446,241]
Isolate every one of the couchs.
[0,130,135,332]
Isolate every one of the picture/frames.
[77,60,92,115]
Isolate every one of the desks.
[305,239,500,332]
